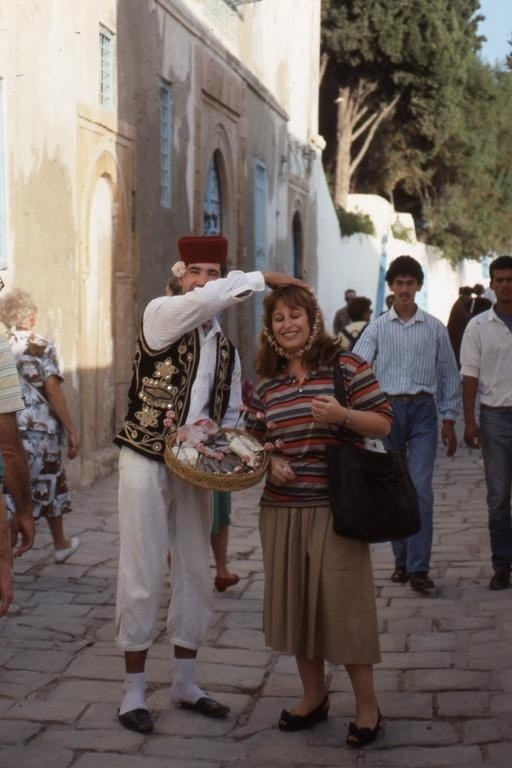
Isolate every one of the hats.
[176,236,230,265]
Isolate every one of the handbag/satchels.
[328,441,424,543]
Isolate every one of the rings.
[314,412,321,419]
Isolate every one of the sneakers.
[487,570,510,591]
[408,573,435,591]
[54,537,81,565]
[390,568,410,584]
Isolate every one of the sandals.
[344,707,383,748]
[276,693,331,732]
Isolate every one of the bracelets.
[342,409,352,428]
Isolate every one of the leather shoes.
[181,692,232,718]
[115,705,156,733]
[214,573,241,593]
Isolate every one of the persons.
[161,282,238,590]
[3,287,82,564]
[117,233,309,735]
[379,294,392,317]
[354,254,462,588]
[334,289,356,337]
[447,256,512,589]
[1,279,34,615]
[337,295,371,350]
[244,285,395,748]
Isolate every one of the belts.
[383,393,433,400]
[480,403,511,413]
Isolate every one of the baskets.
[164,426,271,493]
